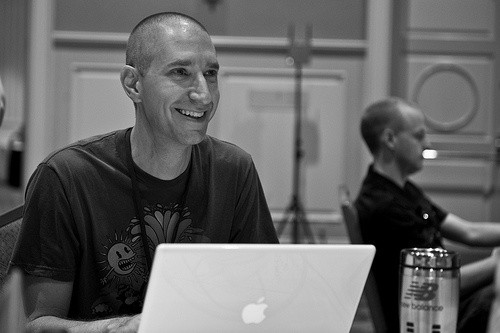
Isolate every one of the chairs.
[338,186,390,333]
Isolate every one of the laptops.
[138,242,377,333]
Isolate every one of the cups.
[397,249,460,333]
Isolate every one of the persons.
[3,12,279,333]
[350,100,500,333]
